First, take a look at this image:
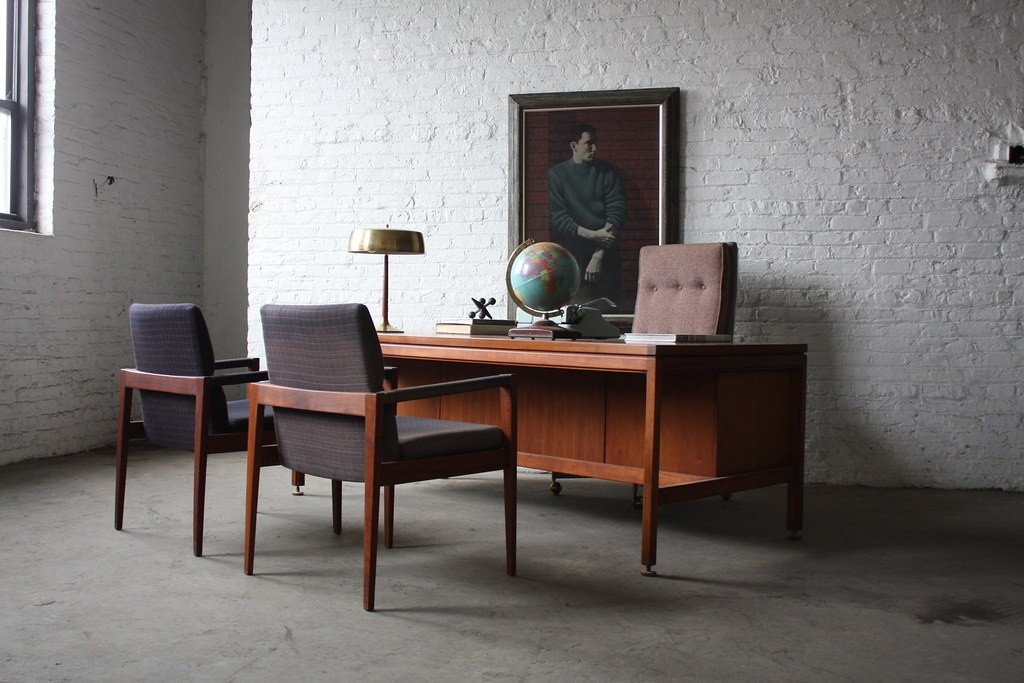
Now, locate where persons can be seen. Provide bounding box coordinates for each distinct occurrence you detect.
[549,124,624,304]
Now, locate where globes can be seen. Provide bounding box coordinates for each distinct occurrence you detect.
[504,236,583,341]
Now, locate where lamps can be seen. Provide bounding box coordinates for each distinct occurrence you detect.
[348,223,425,333]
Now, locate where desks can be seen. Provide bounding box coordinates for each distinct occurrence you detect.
[290,333,808,576]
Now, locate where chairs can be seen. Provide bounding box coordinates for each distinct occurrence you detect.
[244,303,520,611]
[550,242,739,510]
[114,303,342,557]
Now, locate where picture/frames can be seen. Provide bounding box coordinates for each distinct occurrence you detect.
[507,88,681,324]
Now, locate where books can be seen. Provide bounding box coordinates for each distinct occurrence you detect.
[625,333,732,343]
[436,321,519,336]
